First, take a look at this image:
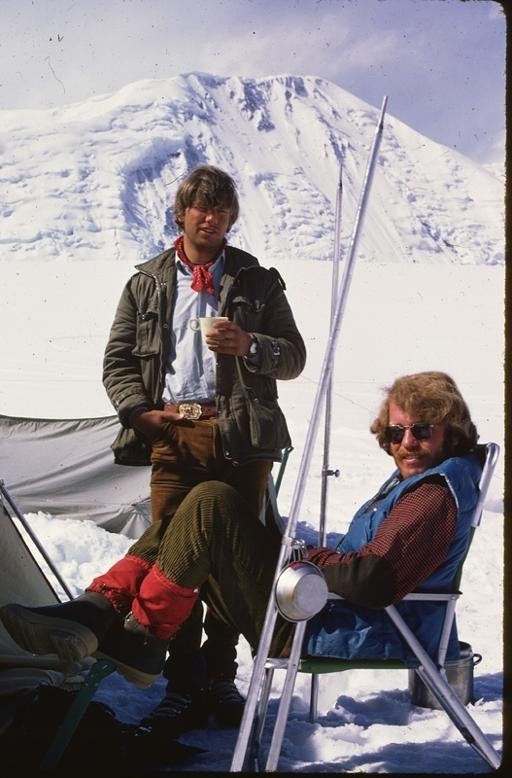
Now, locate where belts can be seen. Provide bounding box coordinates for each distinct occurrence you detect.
[161,402,218,420]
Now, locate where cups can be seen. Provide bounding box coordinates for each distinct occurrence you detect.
[191,316,228,347]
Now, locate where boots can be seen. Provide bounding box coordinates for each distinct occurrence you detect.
[137,660,257,737]
[0,589,171,689]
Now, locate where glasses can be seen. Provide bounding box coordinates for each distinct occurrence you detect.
[385,418,445,443]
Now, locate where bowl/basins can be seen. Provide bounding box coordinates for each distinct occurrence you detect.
[275,560,330,624]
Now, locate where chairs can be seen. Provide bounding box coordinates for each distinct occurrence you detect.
[245,443,504,774]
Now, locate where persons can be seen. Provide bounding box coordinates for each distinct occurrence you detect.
[99,167,311,734]
[1,366,483,689]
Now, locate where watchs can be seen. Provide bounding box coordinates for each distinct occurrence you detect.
[244,333,257,362]
